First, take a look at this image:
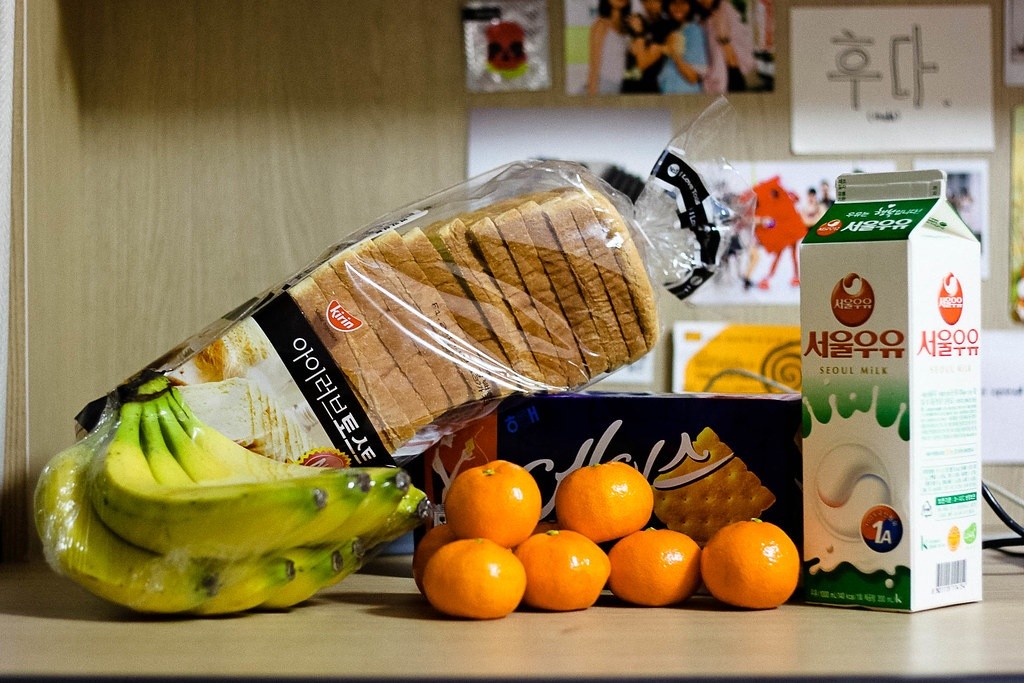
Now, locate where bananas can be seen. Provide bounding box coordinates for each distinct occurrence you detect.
[31,370,433,617]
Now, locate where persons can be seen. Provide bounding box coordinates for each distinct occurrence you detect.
[801,180,834,228]
[589,0,762,95]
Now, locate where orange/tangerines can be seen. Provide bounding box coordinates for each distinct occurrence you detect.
[412,460,800,620]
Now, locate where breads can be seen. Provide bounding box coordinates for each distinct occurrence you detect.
[72,187,662,467]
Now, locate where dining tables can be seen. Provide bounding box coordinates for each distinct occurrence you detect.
[2,463,1022,681]
